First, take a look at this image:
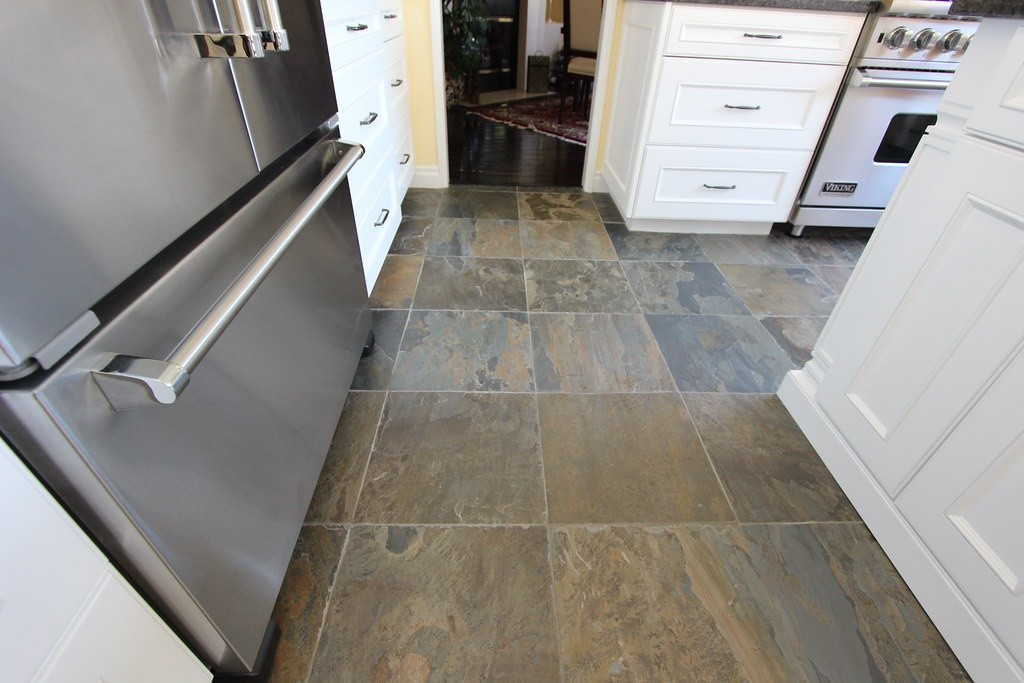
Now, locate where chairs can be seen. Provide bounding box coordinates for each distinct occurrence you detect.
[558,0,604,125]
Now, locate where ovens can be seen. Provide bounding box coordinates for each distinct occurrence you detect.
[799,68,956,212]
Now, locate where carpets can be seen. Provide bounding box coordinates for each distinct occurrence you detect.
[467,94,588,148]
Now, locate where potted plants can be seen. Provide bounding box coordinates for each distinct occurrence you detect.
[441,0,488,112]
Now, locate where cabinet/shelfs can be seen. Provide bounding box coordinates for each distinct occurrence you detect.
[0,436,215,683]
[605,0,869,235]
[321,0,414,297]
[774,17,1024,683]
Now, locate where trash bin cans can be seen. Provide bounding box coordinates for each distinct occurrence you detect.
[528,55,550,92]
